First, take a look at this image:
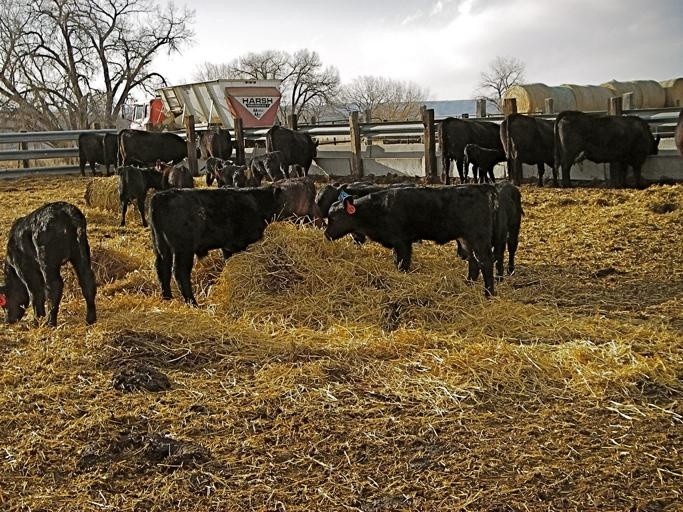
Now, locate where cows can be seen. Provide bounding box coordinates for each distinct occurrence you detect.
[316,178,522,298]
[77,126,325,228]
[437,116,505,185]
[675,109,683,157]
[147,188,312,309]
[463,143,509,185]
[499,112,562,188]
[552,110,661,187]
[1,202,97,326]
